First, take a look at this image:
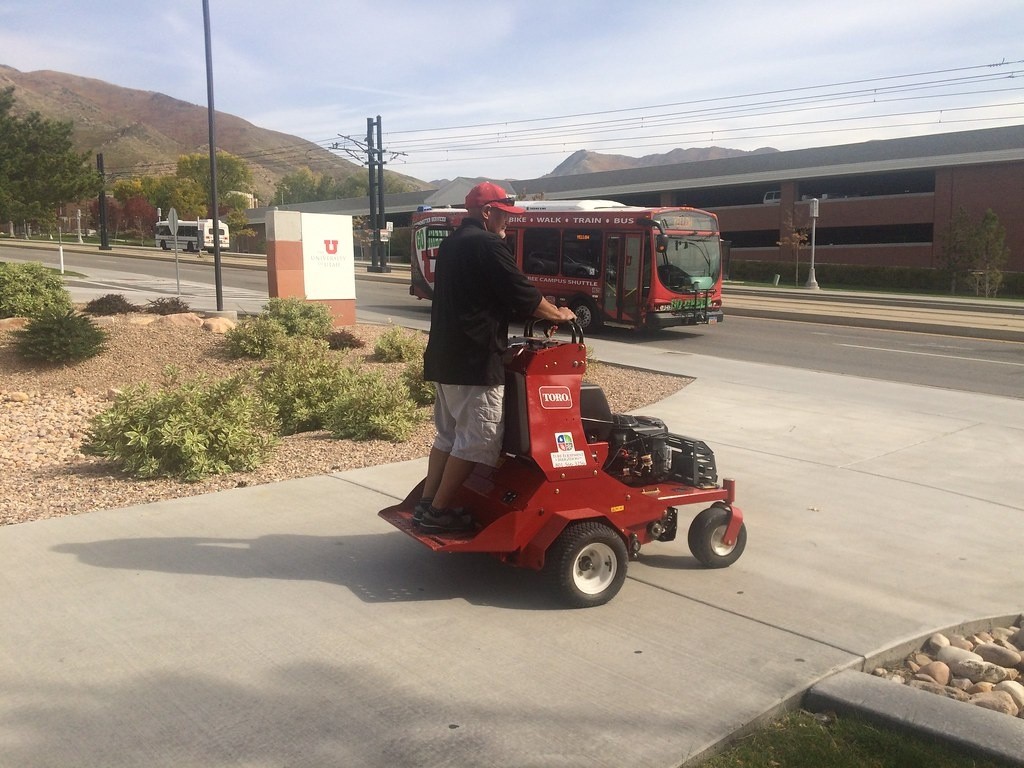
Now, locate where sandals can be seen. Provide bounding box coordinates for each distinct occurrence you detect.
[411,499,432,525]
[418,507,476,532]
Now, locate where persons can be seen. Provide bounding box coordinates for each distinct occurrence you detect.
[413,181,576,533]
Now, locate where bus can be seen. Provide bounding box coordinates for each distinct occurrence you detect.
[155,218,231,251]
[408,198,732,335]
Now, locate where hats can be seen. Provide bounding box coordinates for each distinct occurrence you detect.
[465,181,526,214]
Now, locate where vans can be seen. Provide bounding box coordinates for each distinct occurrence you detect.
[764,190,780,205]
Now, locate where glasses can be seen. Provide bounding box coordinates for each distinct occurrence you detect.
[482,196,516,209]
